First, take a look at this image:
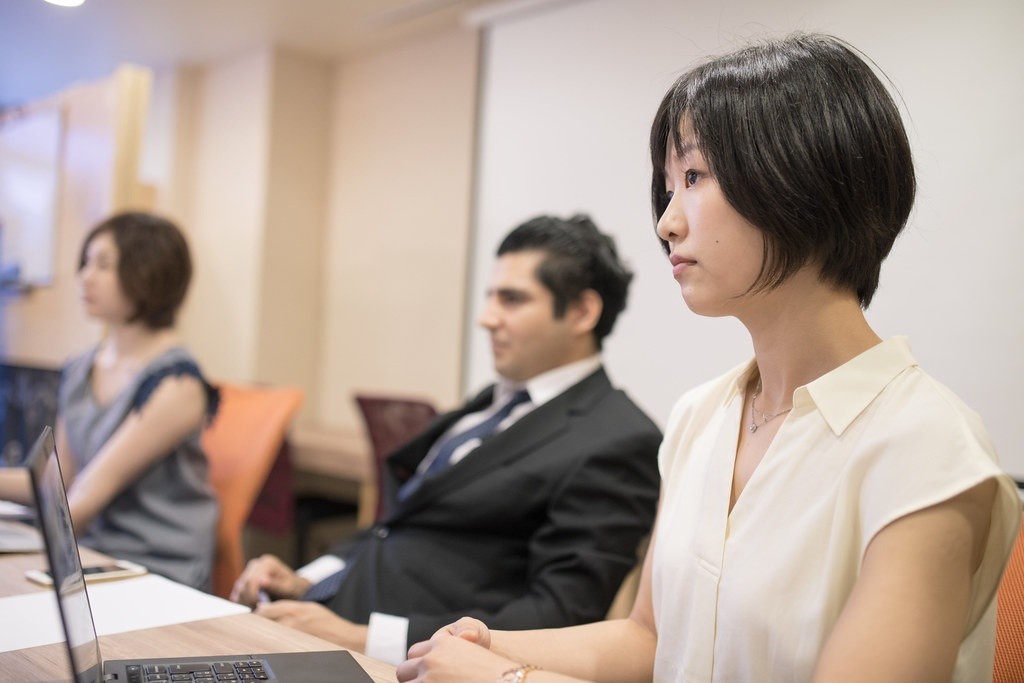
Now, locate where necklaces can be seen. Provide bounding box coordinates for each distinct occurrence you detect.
[750,374,792,434]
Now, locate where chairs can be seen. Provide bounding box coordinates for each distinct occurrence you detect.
[288,369,380,532]
[193,377,303,601]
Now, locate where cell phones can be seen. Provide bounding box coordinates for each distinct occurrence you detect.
[26,560,148,585]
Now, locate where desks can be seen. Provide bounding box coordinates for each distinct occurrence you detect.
[0,523,406,683]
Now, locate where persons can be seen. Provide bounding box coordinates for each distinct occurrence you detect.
[232,35,1019,683]
[0,213,223,588]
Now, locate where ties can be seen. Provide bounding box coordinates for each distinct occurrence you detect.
[301,390,530,601]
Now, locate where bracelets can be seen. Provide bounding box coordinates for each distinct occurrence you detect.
[503,664,543,683]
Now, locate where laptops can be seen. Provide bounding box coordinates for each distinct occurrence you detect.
[26,424,374,683]
